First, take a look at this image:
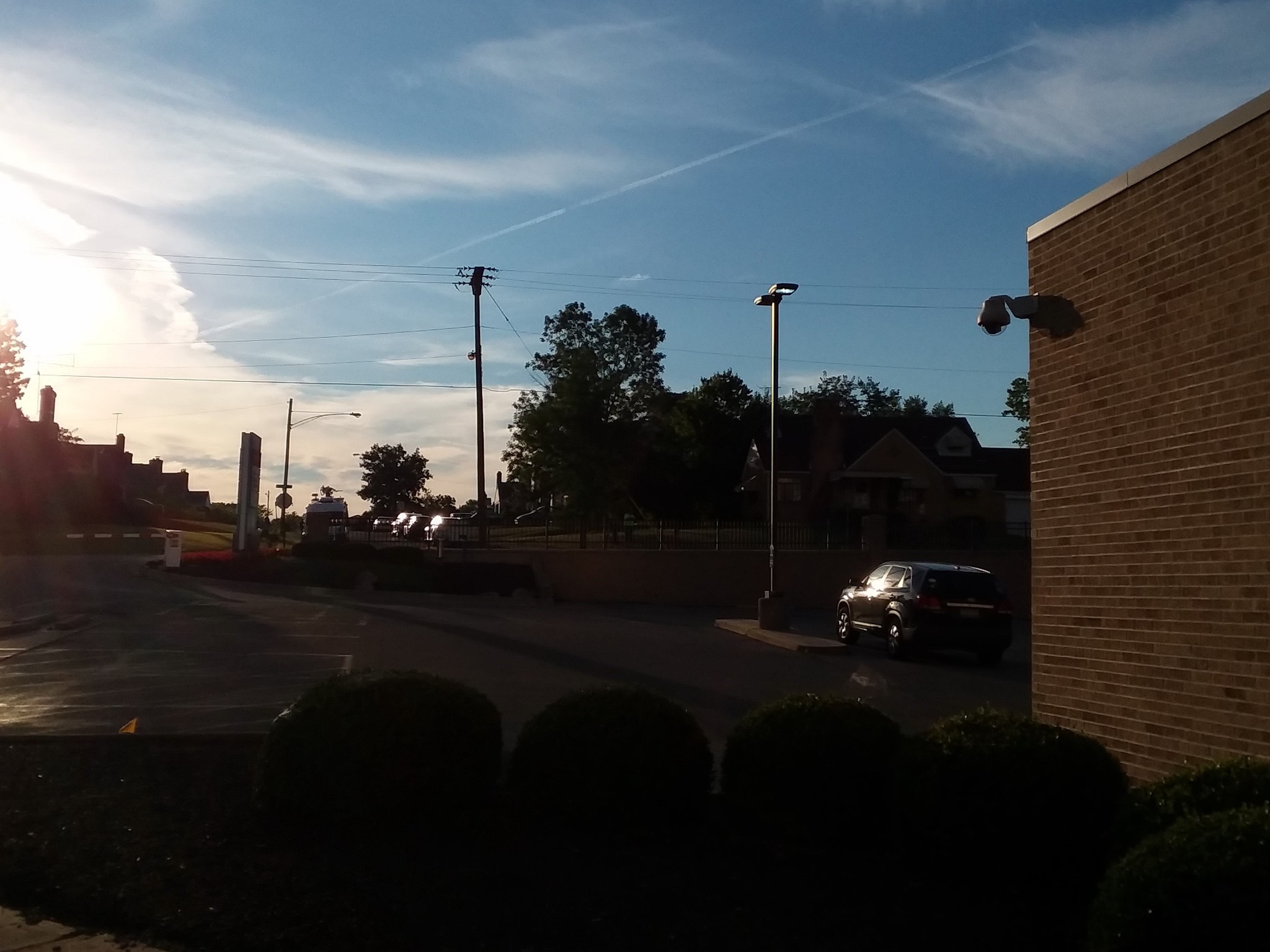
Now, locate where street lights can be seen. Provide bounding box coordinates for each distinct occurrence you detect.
[265,490,269,517]
[753,282,801,631]
[280,398,363,547]
[467,351,489,546]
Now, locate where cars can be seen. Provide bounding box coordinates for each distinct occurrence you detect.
[126,497,165,525]
[513,505,568,526]
[373,512,505,546]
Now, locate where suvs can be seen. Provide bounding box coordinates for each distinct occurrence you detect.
[836,559,1014,667]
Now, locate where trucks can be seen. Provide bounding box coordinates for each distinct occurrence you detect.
[303,498,350,545]
